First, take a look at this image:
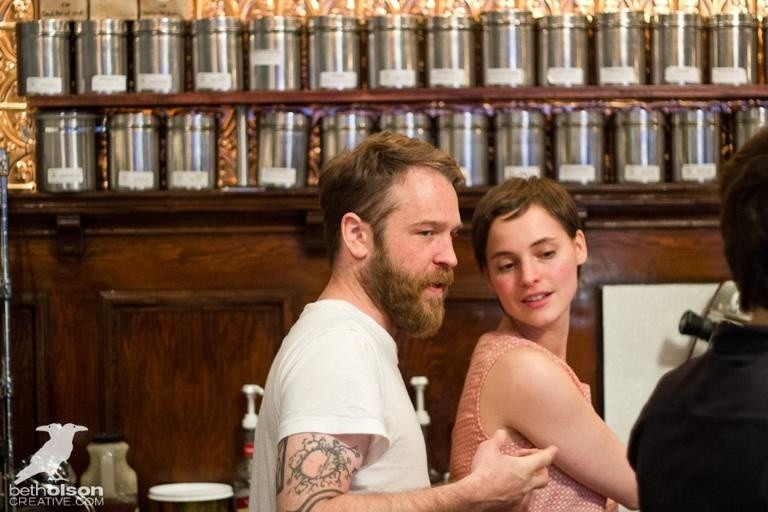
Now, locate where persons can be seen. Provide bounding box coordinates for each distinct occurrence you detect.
[625,113,768,512]
[448,175,638,512]
[249,130,558,512]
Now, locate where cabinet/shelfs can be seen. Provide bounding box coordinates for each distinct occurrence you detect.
[0,201,334,511]
[334,197,733,512]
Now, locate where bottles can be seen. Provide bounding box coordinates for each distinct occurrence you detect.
[232,444,254,512]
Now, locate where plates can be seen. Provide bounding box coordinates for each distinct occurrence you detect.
[148,482,234,497]
[147,493,234,503]
